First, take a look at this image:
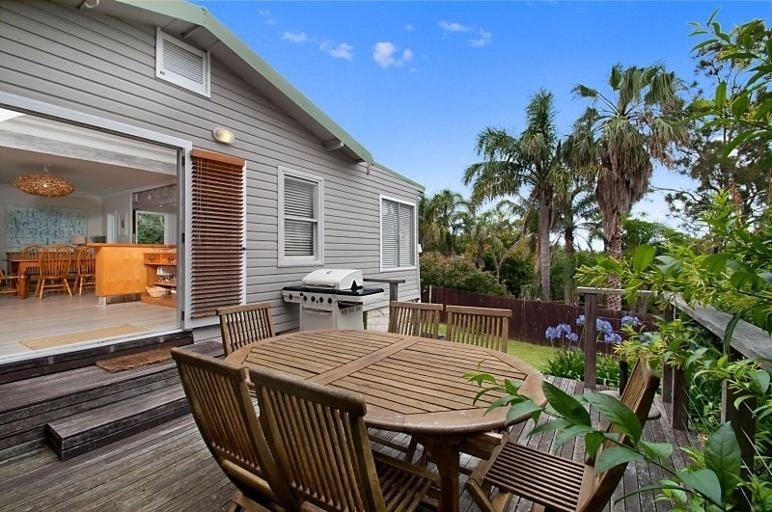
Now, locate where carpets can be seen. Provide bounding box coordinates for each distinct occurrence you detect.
[95,345,180,373]
[21,321,146,351]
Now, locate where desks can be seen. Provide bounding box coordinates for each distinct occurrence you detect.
[220,327,551,511]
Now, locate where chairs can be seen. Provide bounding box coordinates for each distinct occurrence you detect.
[216,301,275,356]
[461,354,661,510]
[0,240,103,303]
[249,365,441,511]
[445,306,512,354]
[170,345,324,512]
[388,300,444,339]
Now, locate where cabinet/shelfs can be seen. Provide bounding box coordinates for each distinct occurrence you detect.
[89,239,176,311]
[142,253,176,307]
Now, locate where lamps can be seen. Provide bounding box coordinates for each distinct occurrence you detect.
[11,163,75,200]
[213,125,237,145]
